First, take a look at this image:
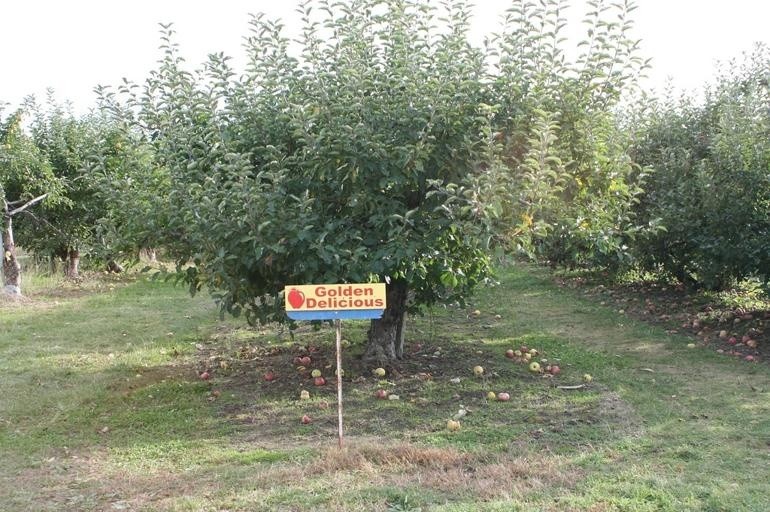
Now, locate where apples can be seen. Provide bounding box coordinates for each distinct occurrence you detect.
[586,284,769,362]
[200,309,592,430]
[289,289,305,309]
